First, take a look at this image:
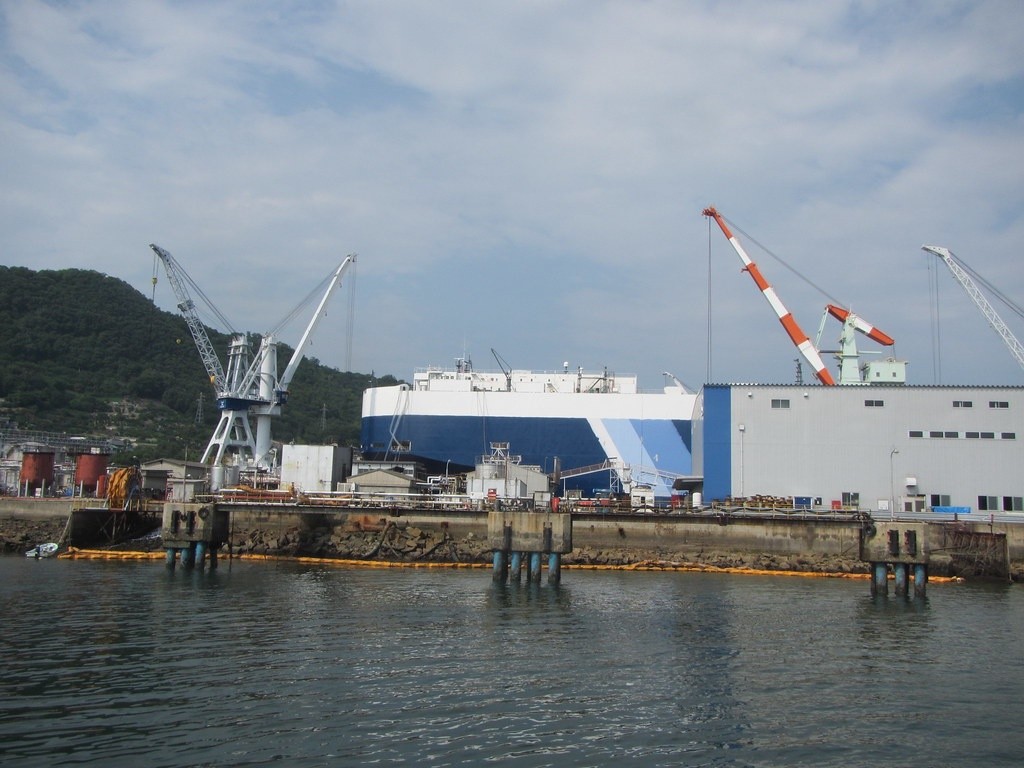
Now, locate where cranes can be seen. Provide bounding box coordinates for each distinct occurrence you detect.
[923,243,1024,385]
[703,206,895,384]
[150,243,358,467]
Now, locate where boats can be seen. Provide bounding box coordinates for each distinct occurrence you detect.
[26,542,58,558]
